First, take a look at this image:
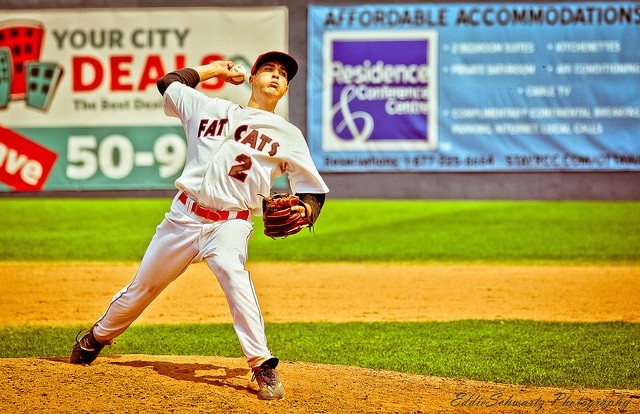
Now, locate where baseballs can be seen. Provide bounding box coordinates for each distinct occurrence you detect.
[230,65,246,83]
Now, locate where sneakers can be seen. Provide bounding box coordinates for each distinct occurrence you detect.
[251,357,285,399]
[70,328,104,364]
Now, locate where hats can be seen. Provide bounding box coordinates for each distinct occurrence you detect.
[251,50,299,81]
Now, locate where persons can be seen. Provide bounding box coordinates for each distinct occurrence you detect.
[70,50,329,400]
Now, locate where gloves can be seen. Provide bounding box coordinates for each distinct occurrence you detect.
[263,198,310,236]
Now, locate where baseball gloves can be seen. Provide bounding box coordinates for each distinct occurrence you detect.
[262,193,309,239]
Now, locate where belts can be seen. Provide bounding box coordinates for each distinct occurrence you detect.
[178,191,249,221]
